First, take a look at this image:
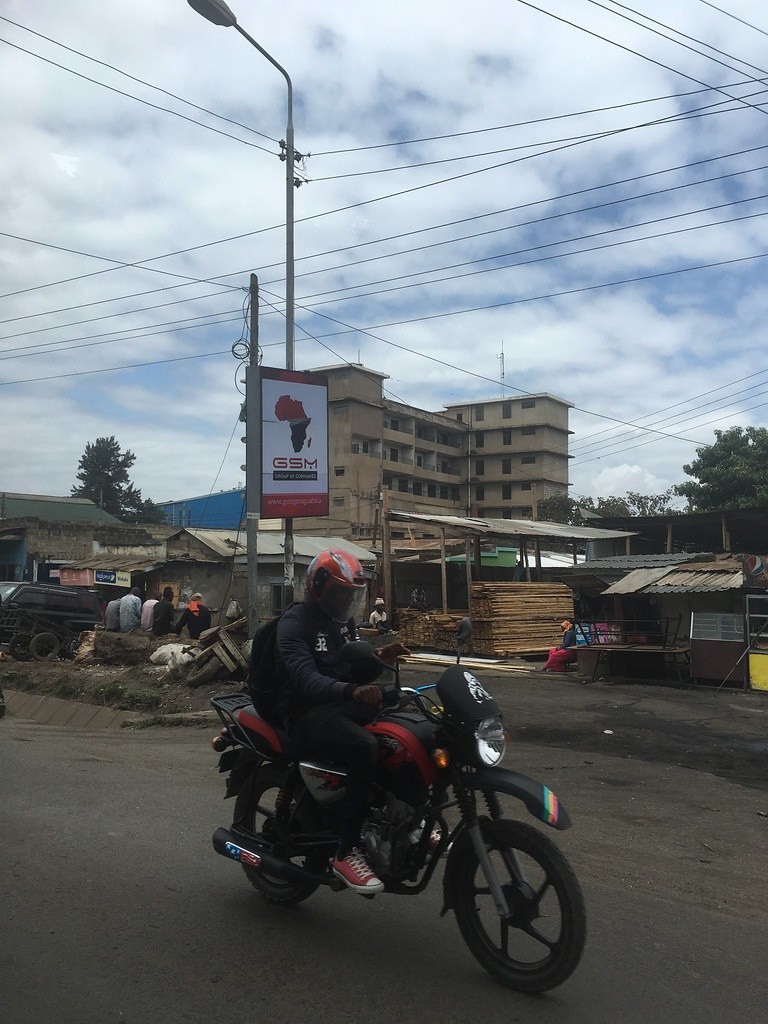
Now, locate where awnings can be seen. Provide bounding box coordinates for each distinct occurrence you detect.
[600,567,744,594]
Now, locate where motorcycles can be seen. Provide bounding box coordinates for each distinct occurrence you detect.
[211,617,587,995]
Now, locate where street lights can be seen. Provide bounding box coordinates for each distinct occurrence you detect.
[187,0,297,613]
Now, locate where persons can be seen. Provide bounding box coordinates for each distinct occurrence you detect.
[120,587,141,630]
[153,587,176,636]
[370,598,386,628]
[141,590,162,630]
[175,593,211,639]
[277,550,455,894]
[106,589,129,631]
[541,621,575,672]
[410,584,432,612]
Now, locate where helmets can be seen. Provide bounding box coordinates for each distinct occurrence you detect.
[304,549,365,603]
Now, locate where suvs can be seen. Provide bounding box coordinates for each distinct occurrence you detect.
[0,580,102,649]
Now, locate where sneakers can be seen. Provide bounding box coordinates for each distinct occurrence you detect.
[332,847,384,894]
[410,827,457,859]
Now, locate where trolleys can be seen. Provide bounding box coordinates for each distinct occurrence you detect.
[0,599,82,663]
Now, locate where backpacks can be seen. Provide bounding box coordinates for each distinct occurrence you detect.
[248,600,305,722]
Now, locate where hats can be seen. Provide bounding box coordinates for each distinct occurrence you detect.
[190,593,203,601]
[374,597,385,606]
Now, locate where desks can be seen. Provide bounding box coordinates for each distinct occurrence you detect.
[564,613,690,683]
